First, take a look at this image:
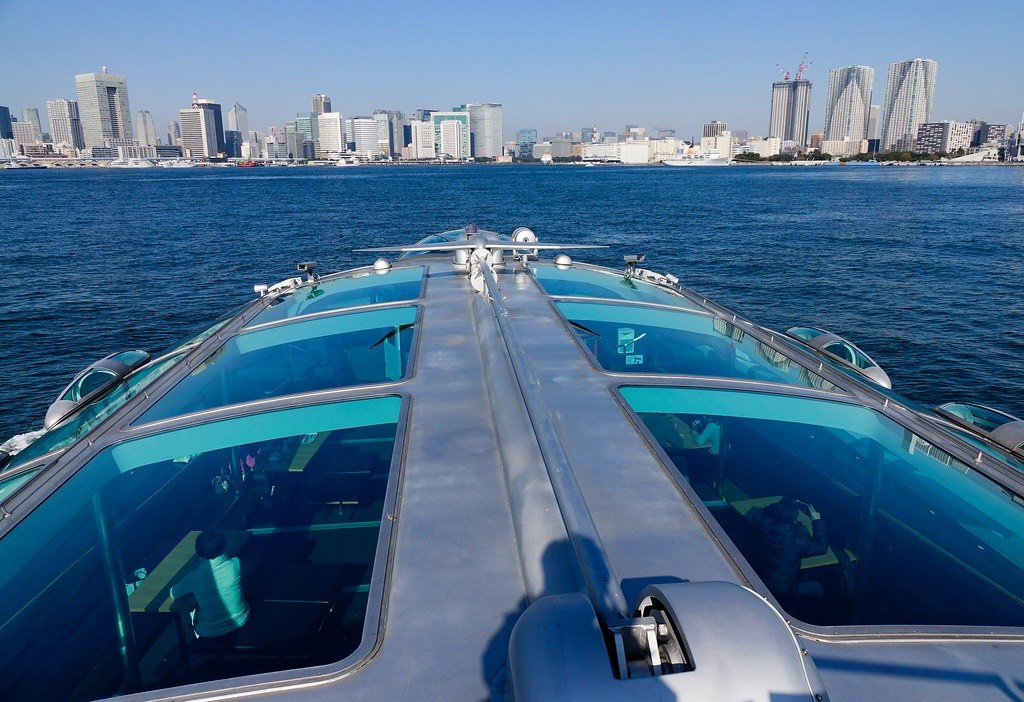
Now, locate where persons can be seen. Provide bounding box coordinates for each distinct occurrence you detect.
[642,413,690,482]
[746,495,830,608]
[692,414,736,481]
[228,443,273,500]
[168,528,253,642]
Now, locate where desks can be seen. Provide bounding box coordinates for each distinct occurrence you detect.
[127,530,201,652]
[728,495,838,570]
[637,412,713,449]
[253,430,333,521]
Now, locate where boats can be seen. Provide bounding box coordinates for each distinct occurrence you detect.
[0,213,1022,702]
[237,161,263,168]
[659,156,733,167]
[104,157,157,168]
[158,160,198,168]
